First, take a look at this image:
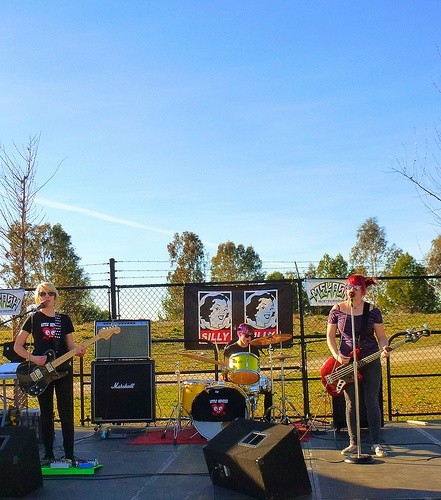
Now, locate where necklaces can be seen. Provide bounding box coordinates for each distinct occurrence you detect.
[354,301,362,309]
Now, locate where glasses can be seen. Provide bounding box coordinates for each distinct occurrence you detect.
[39,291,56,297]
[345,283,363,290]
[240,332,255,339]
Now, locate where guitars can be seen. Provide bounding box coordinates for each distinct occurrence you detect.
[321,324,432,398]
[16,325,121,396]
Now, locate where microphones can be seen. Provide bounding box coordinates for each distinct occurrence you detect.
[348,290,355,298]
[34,301,47,311]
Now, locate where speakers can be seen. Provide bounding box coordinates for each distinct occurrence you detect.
[332,377,384,431]
[0,427,44,498]
[202,417,313,500]
[90,359,155,423]
[93,320,151,360]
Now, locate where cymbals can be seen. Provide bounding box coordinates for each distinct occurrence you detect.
[267,355,299,358]
[179,354,229,367]
[250,334,293,345]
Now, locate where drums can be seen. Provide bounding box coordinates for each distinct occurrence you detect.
[181,379,217,420]
[227,352,260,385]
[191,381,253,441]
[245,376,272,394]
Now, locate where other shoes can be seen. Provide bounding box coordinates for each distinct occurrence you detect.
[41,454,55,460]
[65,456,75,462]
[341,445,356,456]
[372,443,387,457]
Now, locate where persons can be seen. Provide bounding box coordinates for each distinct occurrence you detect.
[327,274,390,458]
[13,282,86,465]
[223,323,273,422]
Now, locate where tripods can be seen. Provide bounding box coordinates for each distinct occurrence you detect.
[259,343,301,434]
[271,343,311,430]
[160,364,194,445]
